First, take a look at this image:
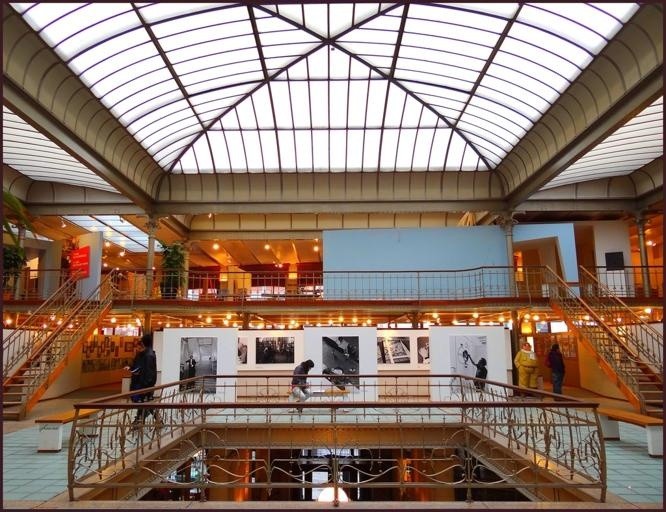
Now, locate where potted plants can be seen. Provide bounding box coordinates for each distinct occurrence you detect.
[148,236,192,299]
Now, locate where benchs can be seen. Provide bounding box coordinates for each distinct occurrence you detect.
[591,407,666,458]
[35,408,104,453]
[539,281,594,298]
[287,388,350,413]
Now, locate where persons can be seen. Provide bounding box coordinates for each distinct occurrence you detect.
[123,340,152,421]
[131,334,167,430]
[289,358,315,414]
[466,353,489,402]
[185,354,196,386]
[548,343,567,402]
[514,341,542,394]
